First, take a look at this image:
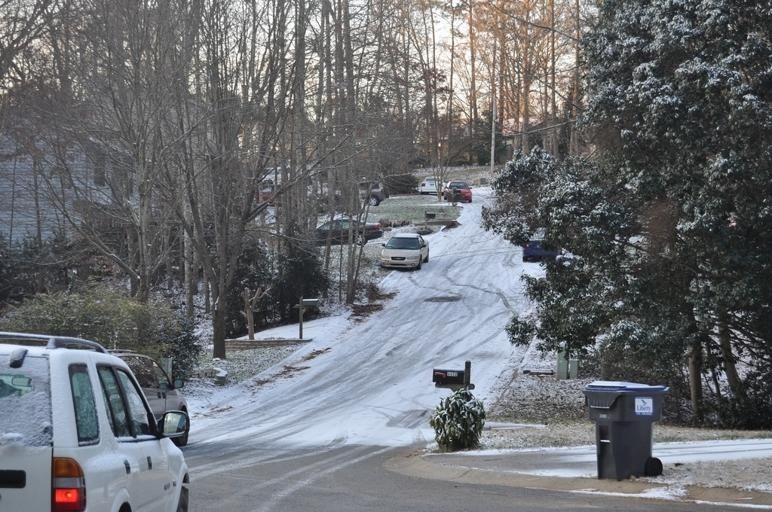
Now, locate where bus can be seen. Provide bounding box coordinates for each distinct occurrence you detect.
[251,166,330,206]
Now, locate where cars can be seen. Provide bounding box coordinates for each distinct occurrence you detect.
[444,180,474,203]
[317,218,384,245]
[381,232,430,270]
[523,226,561,262]
[105,349,190,447]
[420,176,446,196]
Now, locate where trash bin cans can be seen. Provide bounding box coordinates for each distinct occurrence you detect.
[582,380,671,481]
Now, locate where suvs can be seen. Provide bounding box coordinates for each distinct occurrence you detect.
[336,180,387,207]
[0,331,191,512]
